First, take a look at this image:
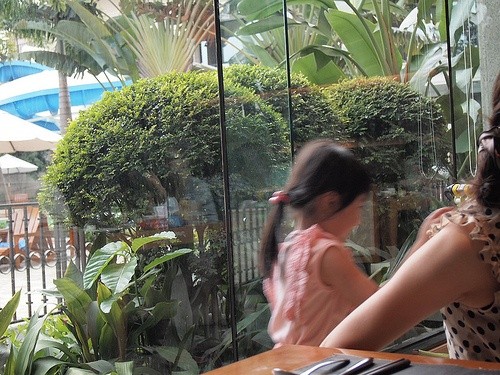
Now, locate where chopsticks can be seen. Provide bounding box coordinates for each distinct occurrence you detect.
[362,357,410,375]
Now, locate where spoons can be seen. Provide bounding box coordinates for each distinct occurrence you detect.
[271,358,350,375]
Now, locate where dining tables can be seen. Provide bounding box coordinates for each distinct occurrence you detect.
[200,344,500,375]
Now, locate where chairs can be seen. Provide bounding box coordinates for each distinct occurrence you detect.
[0,206,55,262]
[12,194,28,212]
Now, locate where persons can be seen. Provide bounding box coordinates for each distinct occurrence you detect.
[258,139,452,348]
[317,72,499,363]
